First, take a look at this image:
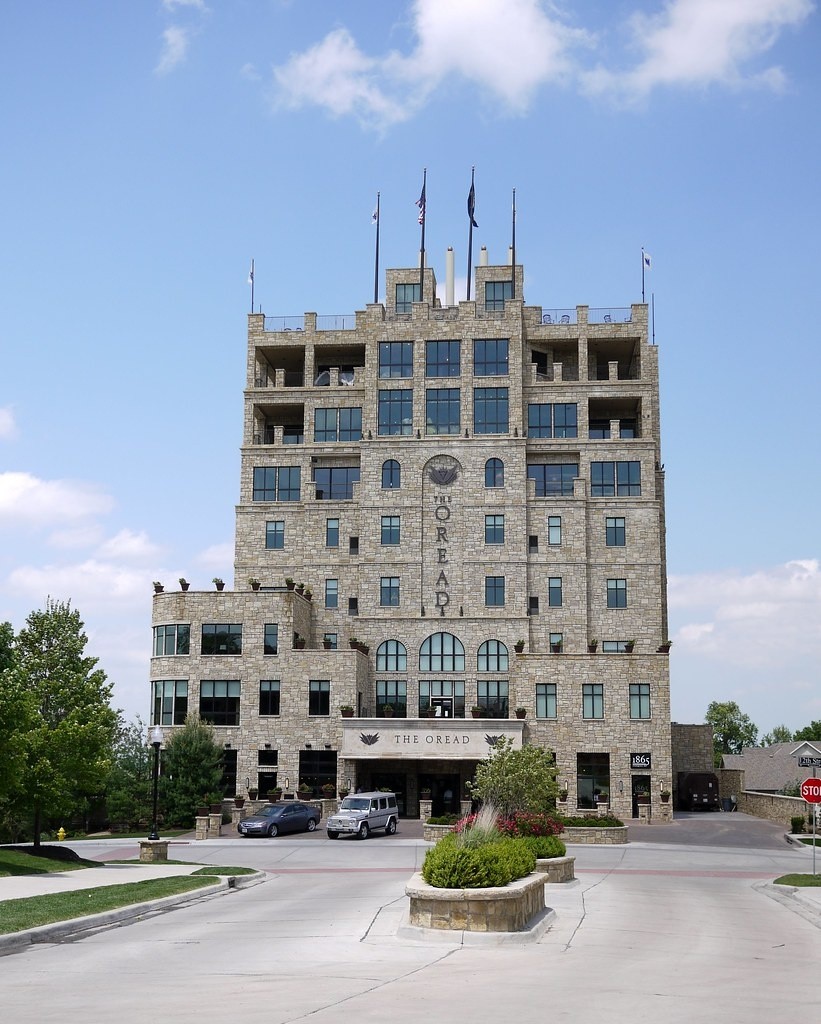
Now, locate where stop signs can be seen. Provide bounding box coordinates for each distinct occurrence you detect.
[801,778,821,804]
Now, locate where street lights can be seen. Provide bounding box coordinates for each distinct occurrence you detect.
[148,724,164,840]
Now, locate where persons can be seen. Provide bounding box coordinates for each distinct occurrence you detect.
[443,785,452,814]
[356,782,380,794]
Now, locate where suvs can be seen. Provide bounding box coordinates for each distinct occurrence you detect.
[688,782,720,812]
[326,792,399,839]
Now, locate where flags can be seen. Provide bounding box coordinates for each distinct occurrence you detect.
[248,262,255,283]
[371,197,378,225]
[415,183,427,224]
[644,252,651,271]
[467,175,479,227]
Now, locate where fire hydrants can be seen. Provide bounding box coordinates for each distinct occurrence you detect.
[56,827,66,841]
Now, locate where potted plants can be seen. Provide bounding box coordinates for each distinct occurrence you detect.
[383,704,394,718]
[463,790,470,801]
[427,706,437,718]
[273,787,283,800]
[339,789,349,800]
[295,583,305,595]
[302,786,313,801]
[285,577,296,590]
[197,802,209,816]
[658,639,673,653]
[660,790,671,802]
[208,791,224,814]
[322,784,336,799]
[267,790,279,803]
[233,794,245,808]
[558,790,569,802]
[248,577,261,591]
[349,637,370,655]
[421,788,431,799]
[321,637,332,649]
[515,707,527,719]
[248,788,259,800]
[552,640,563,653]
[514,640,525,652]
[212,578,226,591]
[178,578,191,591]
[587,639,598,652]
[638,792,650,804]
[296,783,307,799]
[295,637,306,649]
[153,582,164,593]
[623,639,637,653]
[471,706,482,718]
[340,705,354,717]
[598,790,609,803]
[303,589,312,601]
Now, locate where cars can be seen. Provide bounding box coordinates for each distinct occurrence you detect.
[237,802,322,838]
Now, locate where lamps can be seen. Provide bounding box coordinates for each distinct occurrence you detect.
[246,776,249,789]
[660,781,663,792]
[620,781,623,792]
[564,780,568,791]
[286,778,289,789]
[347,779,351,790]
